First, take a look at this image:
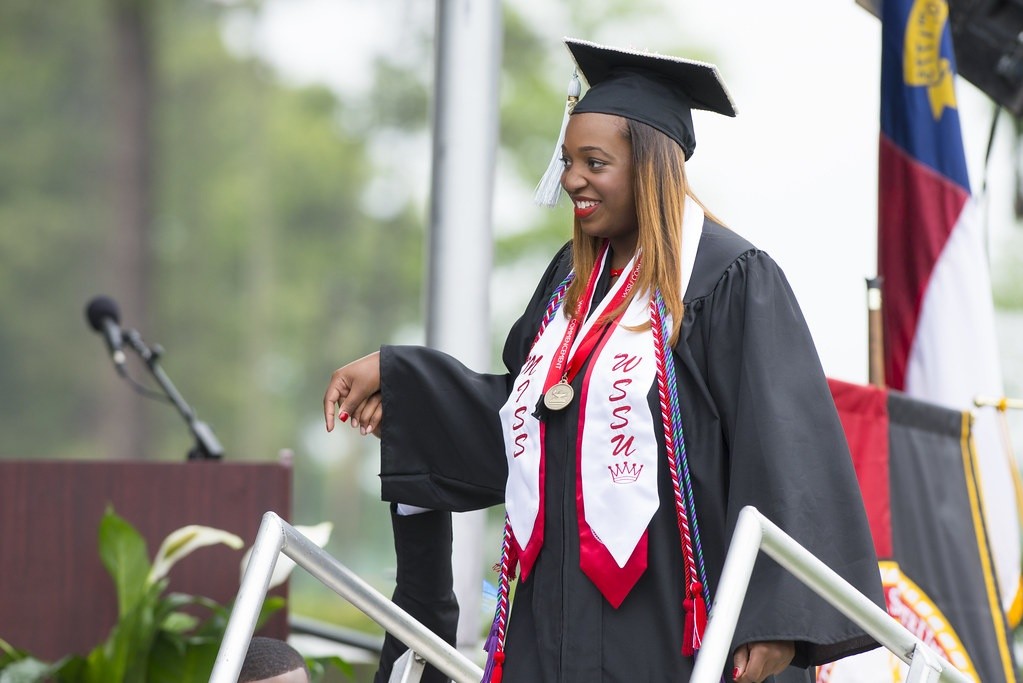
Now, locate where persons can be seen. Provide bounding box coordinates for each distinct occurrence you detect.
[237,391,459,683]
[322,36,888,683]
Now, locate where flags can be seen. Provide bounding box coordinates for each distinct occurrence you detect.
[877,0,1023,629]
[815,377,1023,683]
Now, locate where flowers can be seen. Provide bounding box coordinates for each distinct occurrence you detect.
[0,500,334,683]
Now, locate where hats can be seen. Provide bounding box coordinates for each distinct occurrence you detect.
[533,36,738,208]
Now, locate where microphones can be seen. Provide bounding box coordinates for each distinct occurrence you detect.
[85,294,124,355]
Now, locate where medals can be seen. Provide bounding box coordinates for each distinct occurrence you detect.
[544,383,574,411]
